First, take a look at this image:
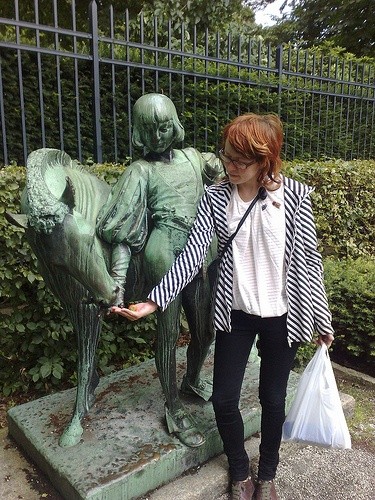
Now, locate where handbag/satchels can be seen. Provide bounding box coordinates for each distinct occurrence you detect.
[206,258,218,295]
[281,342,352,450]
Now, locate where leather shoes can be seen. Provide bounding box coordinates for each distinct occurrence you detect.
[230,478,255,500]
[256,477,278,500]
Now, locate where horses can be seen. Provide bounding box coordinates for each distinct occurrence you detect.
[3,148,219,447]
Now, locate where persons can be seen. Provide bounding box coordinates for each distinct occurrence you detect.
[106,112,335,500]
[95,92,228,447]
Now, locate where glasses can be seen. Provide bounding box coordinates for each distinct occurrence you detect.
[218,147,257,170]
